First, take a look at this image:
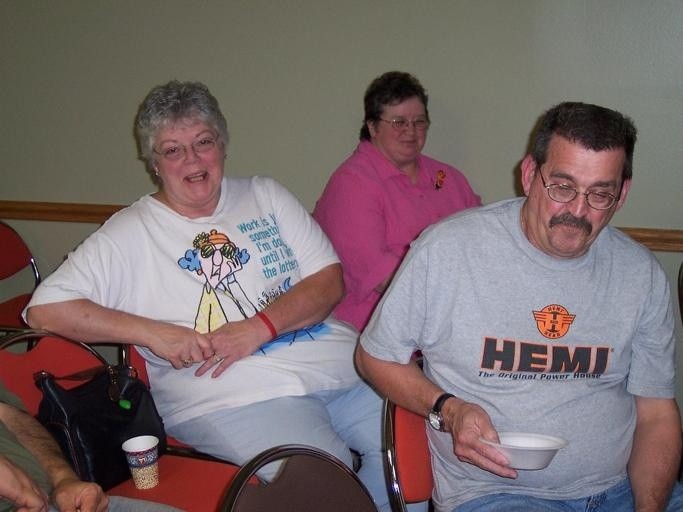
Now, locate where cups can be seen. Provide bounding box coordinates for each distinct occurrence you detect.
[121,434,160,491]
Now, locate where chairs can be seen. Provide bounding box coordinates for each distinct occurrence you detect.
[119,345,211,460]
[0,333,258,512]
[381,358,437,512]
[221,445,379,512]
[0,222,43,352]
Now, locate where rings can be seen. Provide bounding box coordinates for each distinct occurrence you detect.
[182,358,192,364]
[212,353,221,361]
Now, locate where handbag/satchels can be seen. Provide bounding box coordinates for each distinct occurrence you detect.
[32,364,167,493]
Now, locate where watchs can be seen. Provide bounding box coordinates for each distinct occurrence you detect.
[429,392,456,433]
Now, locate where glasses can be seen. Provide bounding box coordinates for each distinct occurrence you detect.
[152,134,219,161]
[537,167,621,211]
[378,117,430,132]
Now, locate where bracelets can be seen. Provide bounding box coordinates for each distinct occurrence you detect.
[254,311,276,343]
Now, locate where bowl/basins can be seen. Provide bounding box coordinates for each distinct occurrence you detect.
[478,431,567,472]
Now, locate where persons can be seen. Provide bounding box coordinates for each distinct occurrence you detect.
[0,385,187,512]
[21,76,429,512]
[300,69,478,330]
[351,99,683,512]
